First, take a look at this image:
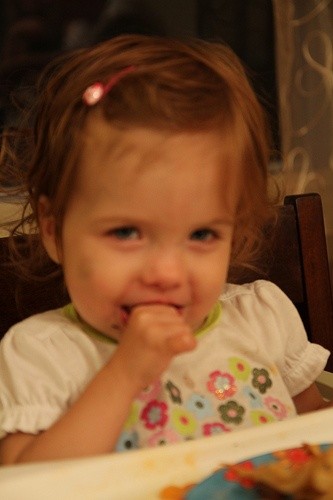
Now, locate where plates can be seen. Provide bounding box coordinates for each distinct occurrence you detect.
[182,443,333,500]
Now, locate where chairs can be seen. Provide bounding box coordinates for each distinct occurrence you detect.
[0,190,333,413]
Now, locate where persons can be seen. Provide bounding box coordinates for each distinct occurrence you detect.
[1,33,333,500]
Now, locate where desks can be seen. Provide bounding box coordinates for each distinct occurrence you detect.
[0,389,332,500]
